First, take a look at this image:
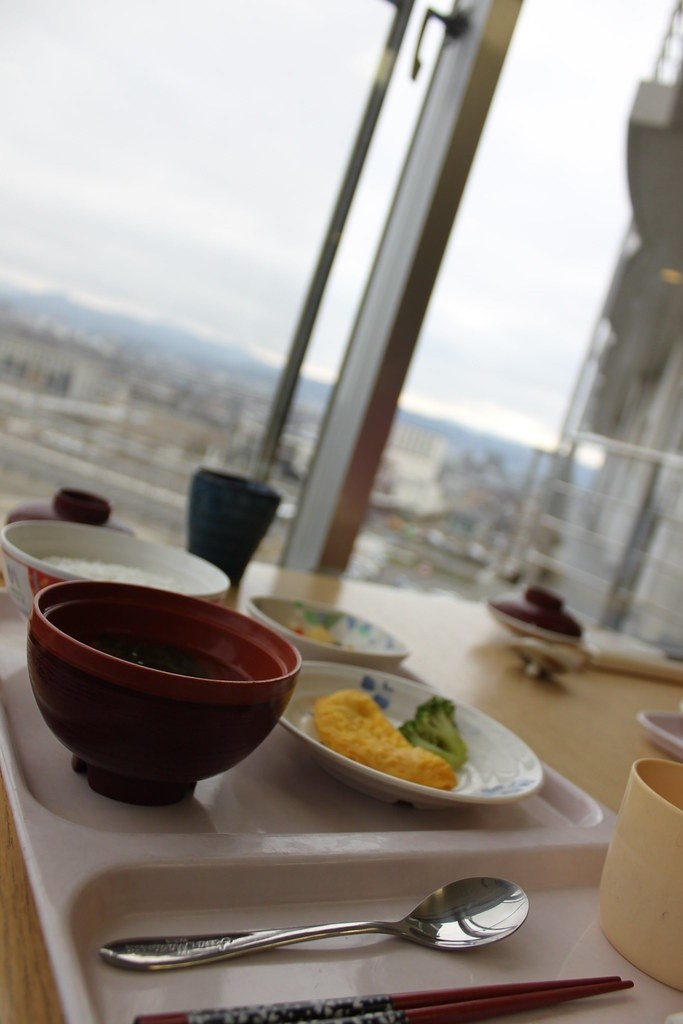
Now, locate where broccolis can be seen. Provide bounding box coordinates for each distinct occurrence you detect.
[400,697,468,768]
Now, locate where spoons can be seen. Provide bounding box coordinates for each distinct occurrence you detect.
[98,875,531,972]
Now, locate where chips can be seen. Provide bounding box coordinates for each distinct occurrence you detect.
[315,690,457,788]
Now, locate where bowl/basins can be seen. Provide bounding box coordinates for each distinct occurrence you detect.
[0,520,233,633]
[246,592,409,674]
[26,579,304,807]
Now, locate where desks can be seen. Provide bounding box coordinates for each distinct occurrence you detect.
[0,551,683,1024]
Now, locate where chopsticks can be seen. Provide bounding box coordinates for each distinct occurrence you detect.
[133,975,636,1024]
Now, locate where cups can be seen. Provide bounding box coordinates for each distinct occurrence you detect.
[597,756,683,993]
[188,464,282,588]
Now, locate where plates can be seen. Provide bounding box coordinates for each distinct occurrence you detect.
[636,708,683,760]
[277,659,546,812]
